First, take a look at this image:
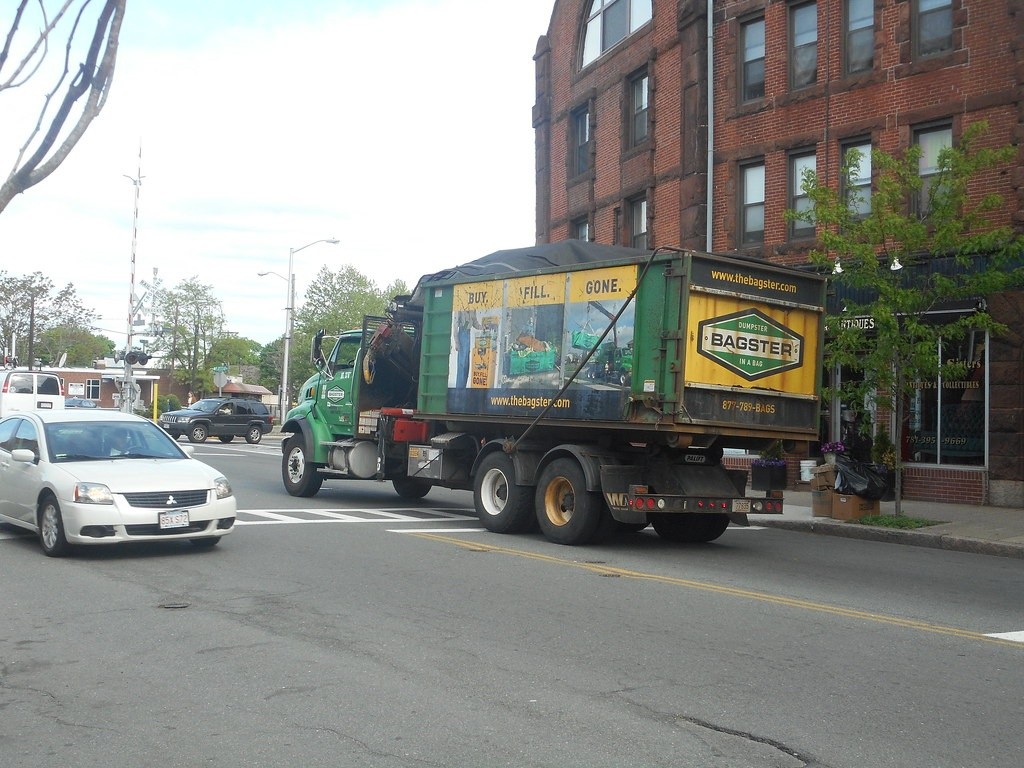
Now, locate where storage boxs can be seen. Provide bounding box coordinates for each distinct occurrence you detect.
[809,466,880,521]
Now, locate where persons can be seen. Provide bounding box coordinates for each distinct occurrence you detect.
[109,428,127,456]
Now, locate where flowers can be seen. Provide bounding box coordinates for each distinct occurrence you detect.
[751,459,787,468]
[821,441,846,453]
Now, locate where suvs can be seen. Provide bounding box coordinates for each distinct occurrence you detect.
[157,398,273,445]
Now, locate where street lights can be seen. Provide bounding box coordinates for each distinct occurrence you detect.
[258,239,339,424]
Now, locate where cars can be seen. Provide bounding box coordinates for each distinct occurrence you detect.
[1,367,65,420]
[0,412,238,557]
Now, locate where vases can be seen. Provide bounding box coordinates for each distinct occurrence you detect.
[825,453,835,464]
[752,466,788,491]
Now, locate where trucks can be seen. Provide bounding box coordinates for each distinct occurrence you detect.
[281,235,821,544]
